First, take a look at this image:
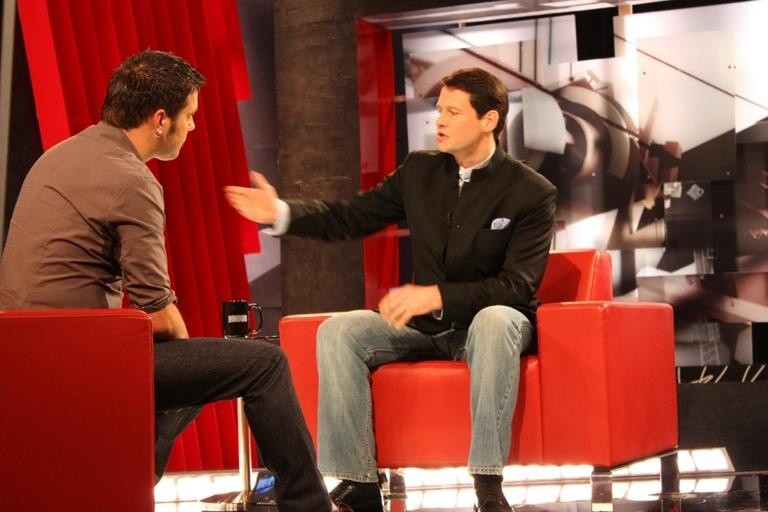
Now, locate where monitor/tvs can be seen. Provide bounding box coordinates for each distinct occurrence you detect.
[390,1,768,388]
[249,469,278,506]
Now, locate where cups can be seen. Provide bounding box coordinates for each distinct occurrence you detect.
[222,299,264,340]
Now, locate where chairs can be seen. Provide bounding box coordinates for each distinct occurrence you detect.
[275,250,685,510]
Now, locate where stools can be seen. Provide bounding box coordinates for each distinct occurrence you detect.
[0,300,158,512]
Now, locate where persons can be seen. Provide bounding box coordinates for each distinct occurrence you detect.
[0,49,334,511]
[223,65,558,512]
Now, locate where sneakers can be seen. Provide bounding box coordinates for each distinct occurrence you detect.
[329,477,383,512]
[471,483,514,512]
